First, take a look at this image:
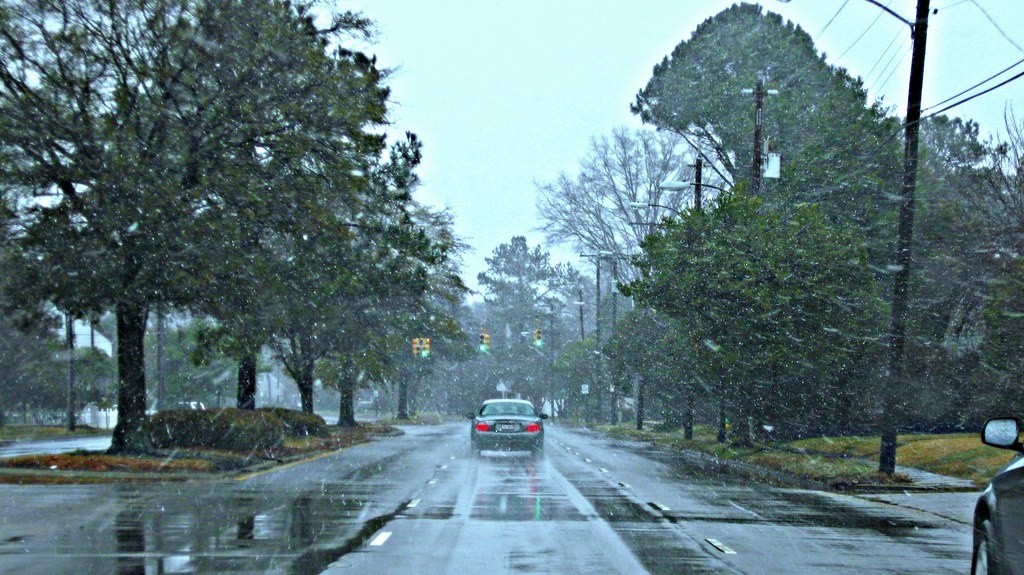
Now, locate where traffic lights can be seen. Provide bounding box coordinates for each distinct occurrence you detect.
[412,339,419,356]
[532,329,542,348]
[483,334,491,350]
[479,335,485,352]
[416,339,427,358]
[424,339,430,356]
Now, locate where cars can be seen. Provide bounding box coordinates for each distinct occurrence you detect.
[970,415,1024,575]
[465,398,548,460]
